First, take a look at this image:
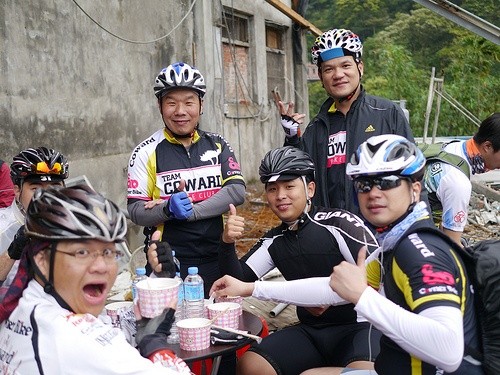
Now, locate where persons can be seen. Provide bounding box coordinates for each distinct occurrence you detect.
[208,134,481,375]
[213,146,380,375]
[0,160,15,208]
[0,147,70,288]
[0,184,195,375]
[277,29,416,232]
[414,110,500,250]
[127,62,246,299]
[460,240,500,375]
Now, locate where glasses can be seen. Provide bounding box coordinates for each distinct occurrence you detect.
[352,174,409,193]
[45,246,124,262]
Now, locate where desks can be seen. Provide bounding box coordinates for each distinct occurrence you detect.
[105,299,263,375]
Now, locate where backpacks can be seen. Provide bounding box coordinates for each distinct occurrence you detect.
[384,222,500,375]
[416,140,470,179]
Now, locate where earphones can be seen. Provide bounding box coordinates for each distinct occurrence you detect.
[412,191,417,202]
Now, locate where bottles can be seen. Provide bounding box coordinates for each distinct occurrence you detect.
[133,268,148,302]
[172,250,180,277]
[184,267,204,319]
[166,272,184,344]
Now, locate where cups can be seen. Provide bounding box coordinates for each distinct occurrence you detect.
[203,298,213,318]
[176,319,211,350]
[208,303,241,331]
[215,295,242,315]
[105,302,132,321]
[136,278,179,318]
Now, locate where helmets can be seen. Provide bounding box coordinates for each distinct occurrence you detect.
[259,146,316,176]
[25,185,128,242]
[346,134,427,175]
[10,147,69,178]
[153,63,206,98]
[312,28,363,63]
